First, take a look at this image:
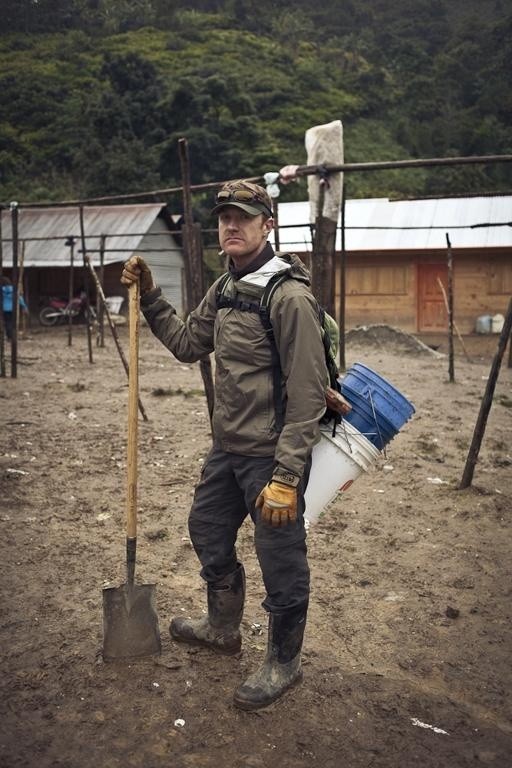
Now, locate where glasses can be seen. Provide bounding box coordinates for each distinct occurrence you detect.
[216,190,254,203]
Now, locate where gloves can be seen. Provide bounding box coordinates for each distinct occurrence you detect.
[254,481,297,528]
[119,255,153,297]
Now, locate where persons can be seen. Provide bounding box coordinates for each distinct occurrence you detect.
[2,275,30,343]
[119,180,331,710]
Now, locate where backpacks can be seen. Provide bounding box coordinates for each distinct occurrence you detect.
[214,252,342,425]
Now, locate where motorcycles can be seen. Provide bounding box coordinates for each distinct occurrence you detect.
[38,292,96,326]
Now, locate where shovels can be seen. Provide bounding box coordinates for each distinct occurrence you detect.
[102,267,161,659]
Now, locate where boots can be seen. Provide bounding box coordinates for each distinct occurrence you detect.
[232,602,308,711]
[168,562,244,655]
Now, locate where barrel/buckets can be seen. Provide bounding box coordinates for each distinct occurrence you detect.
[107,296,125,314]
[340,362,418,454]
[302,414,380,531]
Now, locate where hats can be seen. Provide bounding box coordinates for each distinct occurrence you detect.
[209,180,272,218]
